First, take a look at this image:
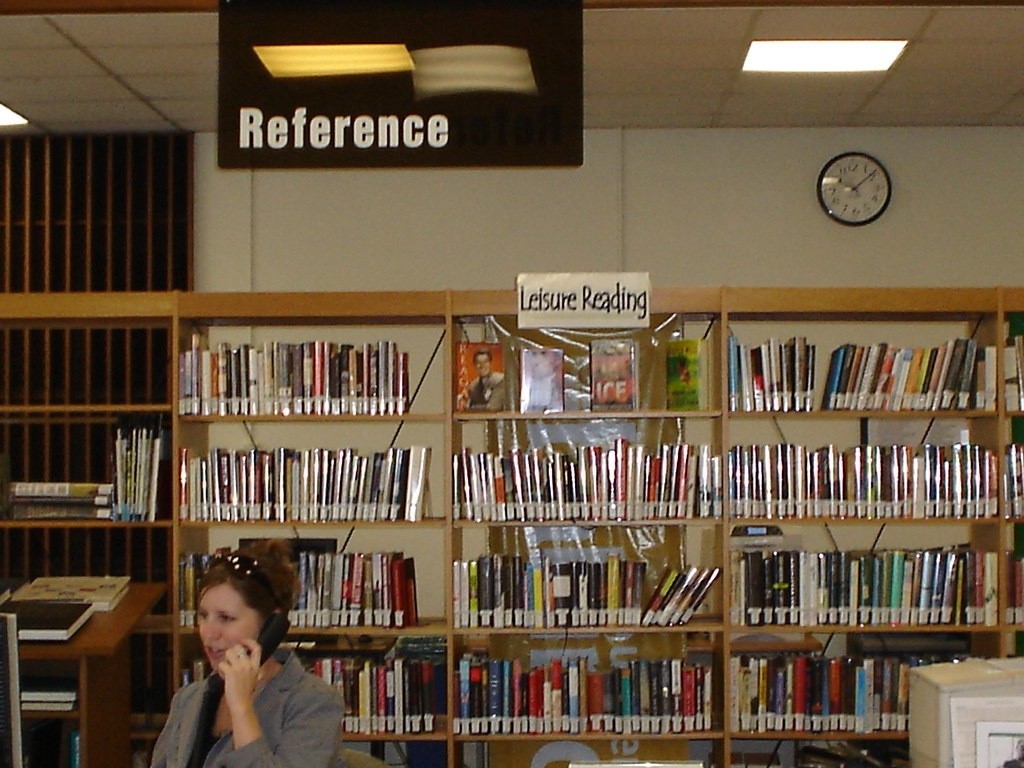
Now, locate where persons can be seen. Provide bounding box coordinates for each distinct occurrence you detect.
[458,347,505,410]
[146,534,345,768]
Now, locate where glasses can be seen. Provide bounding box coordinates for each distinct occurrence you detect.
[206,553,275,599]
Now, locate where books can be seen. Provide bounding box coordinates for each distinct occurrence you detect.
[0,572,133,641]
[9,417,165,522]
[451,434,721,521]
[177,331,413,417]
[457,633,718,735]
[729,522,1024,626]
[347,741,492,768]
[178,442,431,521]
[725,315,1023,414]
[181,646,448,734]
[730,435,1023,518]
[730,629,981,768]
[455,333,712,412]
[16,674,80,767]
[177,535,420,628]
[454,549,724,626]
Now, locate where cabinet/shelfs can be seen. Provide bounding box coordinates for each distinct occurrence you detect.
[0,580,168,768]
[2,287,1024,768]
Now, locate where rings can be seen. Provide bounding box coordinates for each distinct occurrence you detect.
[238,648,247,659]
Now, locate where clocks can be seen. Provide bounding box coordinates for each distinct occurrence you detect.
[817,152,892,225]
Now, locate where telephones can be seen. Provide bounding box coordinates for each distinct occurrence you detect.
[209,613,291,694]
